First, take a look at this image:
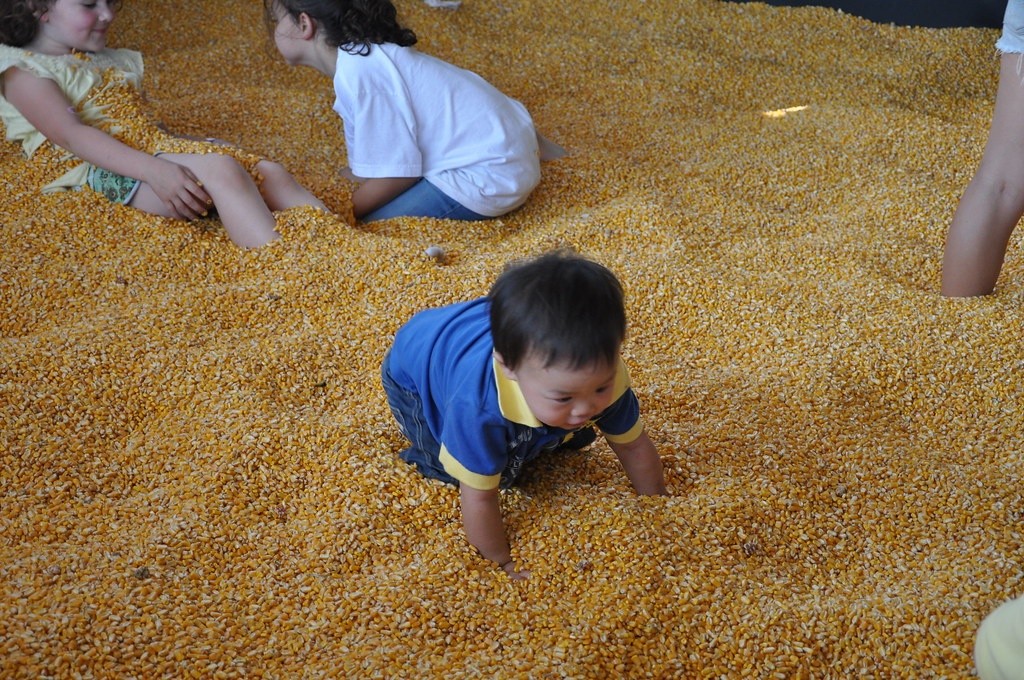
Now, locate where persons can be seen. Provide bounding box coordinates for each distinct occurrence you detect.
[0,0,333,250]
[381,253,670,578]
[262,0,542,226]
[938,0,1024,298]
